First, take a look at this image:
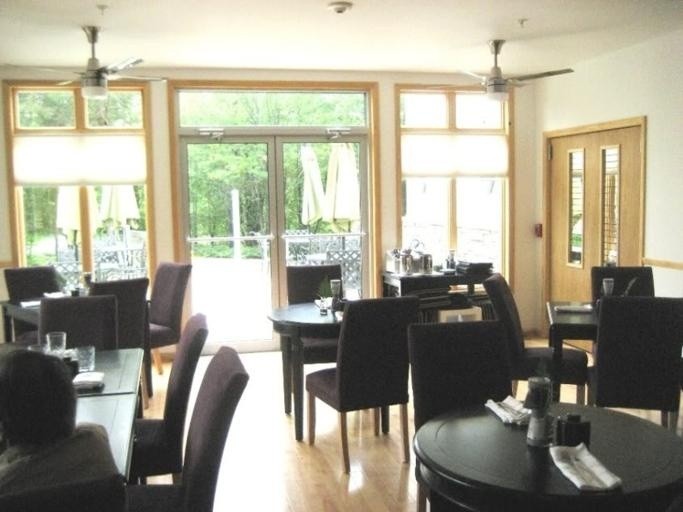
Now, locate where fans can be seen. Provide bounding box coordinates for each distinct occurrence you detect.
[426,38,574,91]
[34,25,166,87]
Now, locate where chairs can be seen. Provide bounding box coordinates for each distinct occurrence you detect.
[42,294,118,350]
[131,317,207,485]
[84,275,149,382]
[149,264,190,396]
[5,267,59,342]
[125,346,250,512]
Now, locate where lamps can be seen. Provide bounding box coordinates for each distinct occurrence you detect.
[81,76,109,100]
[486,84,509,99]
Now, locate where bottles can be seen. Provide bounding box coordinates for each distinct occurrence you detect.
[320,295,328,317]
[596,278,615,319]
[444,249,456,274]
[556,411,592,451]
[330,280,343,313]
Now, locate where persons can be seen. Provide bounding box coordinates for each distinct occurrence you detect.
[0,349,118,498]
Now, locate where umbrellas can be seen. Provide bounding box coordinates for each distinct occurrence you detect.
[55,185,101,264]
[101,183,139,242]
[324,144,360,232]
[300,141,324,224]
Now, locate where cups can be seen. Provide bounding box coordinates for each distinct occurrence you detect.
[28,331,96,372]
